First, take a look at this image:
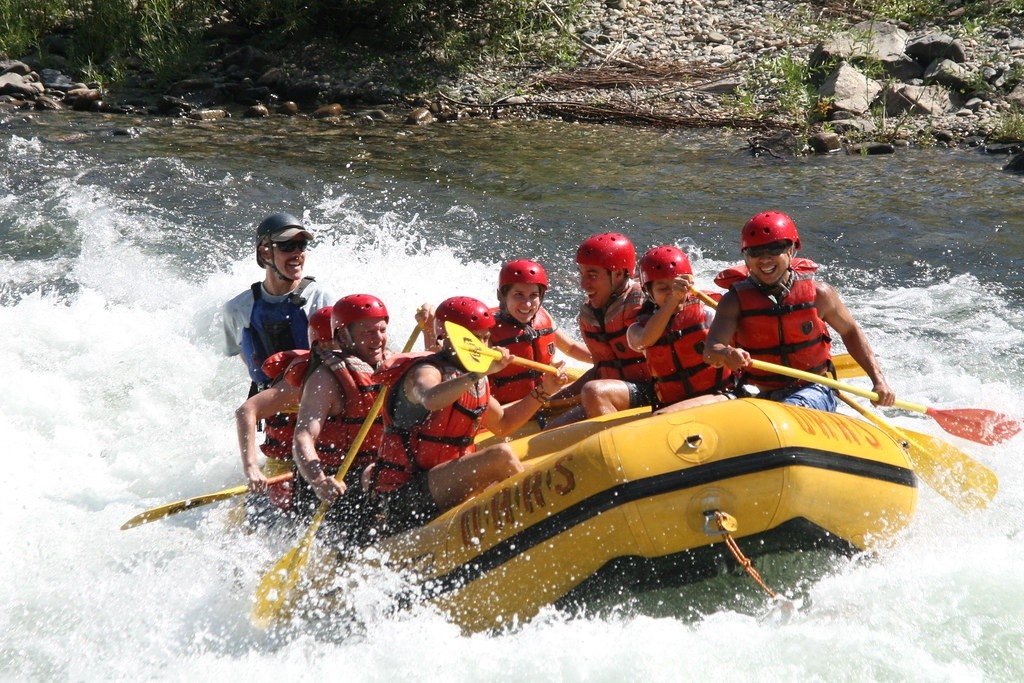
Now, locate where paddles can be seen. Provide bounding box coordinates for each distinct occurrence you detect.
[675,273,998,513]
[544,352,869,409]
[121,469,297,533]
[745,357,1024,446]
[443,321,587,382]
[250,308,428,633]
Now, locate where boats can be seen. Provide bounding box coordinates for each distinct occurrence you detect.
[223,396,918,635]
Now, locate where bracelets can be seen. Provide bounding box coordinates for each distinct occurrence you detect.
[469,371,482,384]
[530,383,551,406]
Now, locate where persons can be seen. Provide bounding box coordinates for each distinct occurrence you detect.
[702,211,895,413]
[235,232,741,552]
[221,211,338,392]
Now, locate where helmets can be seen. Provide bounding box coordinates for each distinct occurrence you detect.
[256,213,314,269]
[639,245,694,293]
[309,306,334,345]
[740,209,802,255]
[331,294,389,340]
[434,296,496,341]
[499,258,547,290]
[576,232,636,277]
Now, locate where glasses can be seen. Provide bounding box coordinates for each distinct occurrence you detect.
[265,240,308,252]
[743,242,791,256]
